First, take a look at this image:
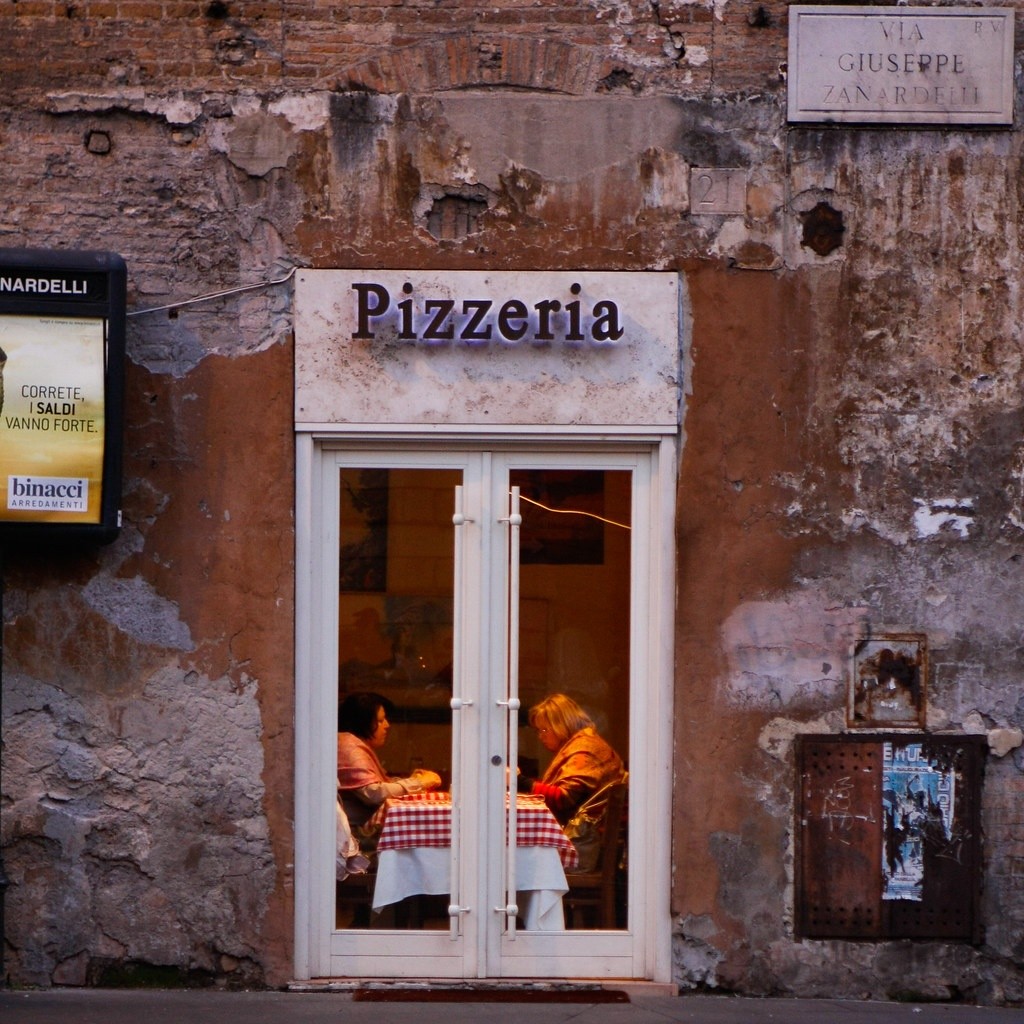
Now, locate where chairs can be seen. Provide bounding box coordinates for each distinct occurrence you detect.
[565,783,629,929]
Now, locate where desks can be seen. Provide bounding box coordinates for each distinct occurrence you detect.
[362,789,578,932]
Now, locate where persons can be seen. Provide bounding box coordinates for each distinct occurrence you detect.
[338,694,442,807]
[505,693,623,826]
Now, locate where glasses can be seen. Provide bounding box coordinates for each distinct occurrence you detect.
[535,727,554,736]
